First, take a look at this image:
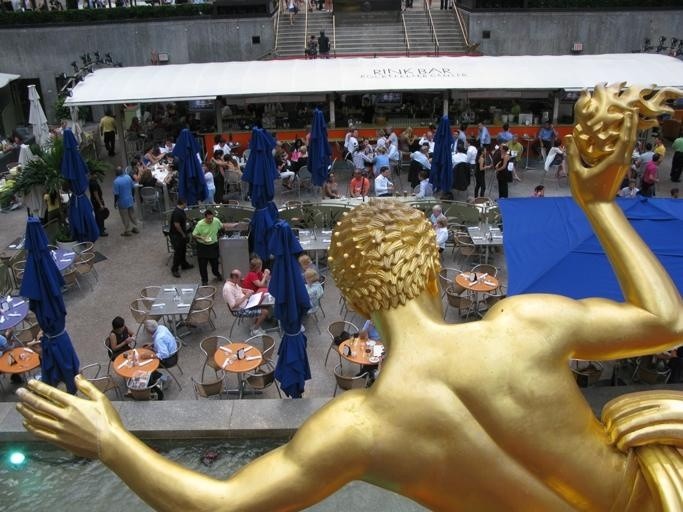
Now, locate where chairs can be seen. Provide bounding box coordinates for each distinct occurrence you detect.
[324,295,385,400]
[1,99,681,259]
[79,362,124,400]
[229,275,325,338]
[190,335,282,400]
[157,342,183,391]
[125,372,164,403]
[2,242,98,402]
[130,285,216,346]
[438,265,507,324]
[570,346,681,388]
[102,337,136,374]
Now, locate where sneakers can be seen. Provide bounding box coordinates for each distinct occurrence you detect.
[163,376,173,390]
[108,152,115,156]
[10,200,22,210]
[250,324,266,337]
[182,264,193,270]
[171,268,181,278]
[203,275,222,287]
[121,231,131,236]
[132,228,140,233]
[100,232,109,237]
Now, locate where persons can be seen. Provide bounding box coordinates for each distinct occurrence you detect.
[11,0,161,11]
[616,132,683,198]
[42,105,523,402]
[15,107,683,511]
[658,348,682,384]
[281,0,333,59]
[531,120,566,197]
[405,0,448,10]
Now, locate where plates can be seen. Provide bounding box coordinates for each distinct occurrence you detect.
[366,339,376,345]
[368,356,380,363]
